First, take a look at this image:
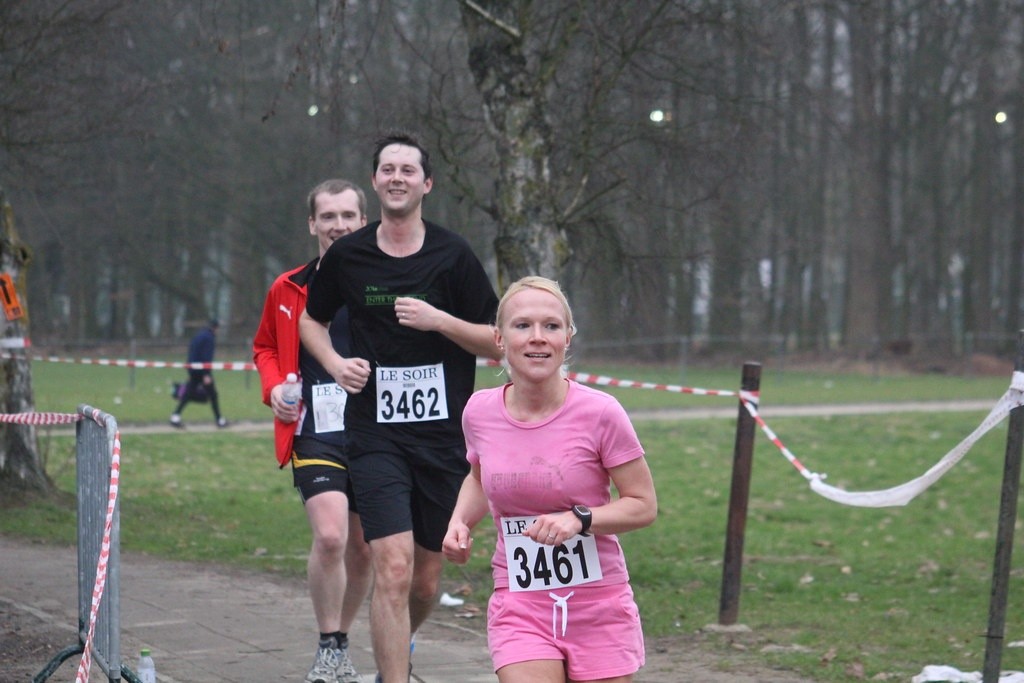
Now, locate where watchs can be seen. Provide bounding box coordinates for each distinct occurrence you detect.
[573,504,592,537]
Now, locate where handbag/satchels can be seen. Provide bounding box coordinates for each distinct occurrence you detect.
[171,381,207,402]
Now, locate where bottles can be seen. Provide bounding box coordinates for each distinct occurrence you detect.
[137,649,155,683]
[283,373,300,423]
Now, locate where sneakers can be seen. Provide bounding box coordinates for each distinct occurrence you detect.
[337,639,364,683]
[304,637,339,683]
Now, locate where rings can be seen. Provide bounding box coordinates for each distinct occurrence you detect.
[548,535,555,539]
[402,312,404,319]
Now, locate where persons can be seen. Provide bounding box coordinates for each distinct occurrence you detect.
[169,318,232,429]
[253,179,367,683]
[298,135,506,683]
[441,276,658,683]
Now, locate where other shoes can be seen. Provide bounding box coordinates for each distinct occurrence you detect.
[170,415,185,428]
[218,419,232,428]
[375,642,424,683]
